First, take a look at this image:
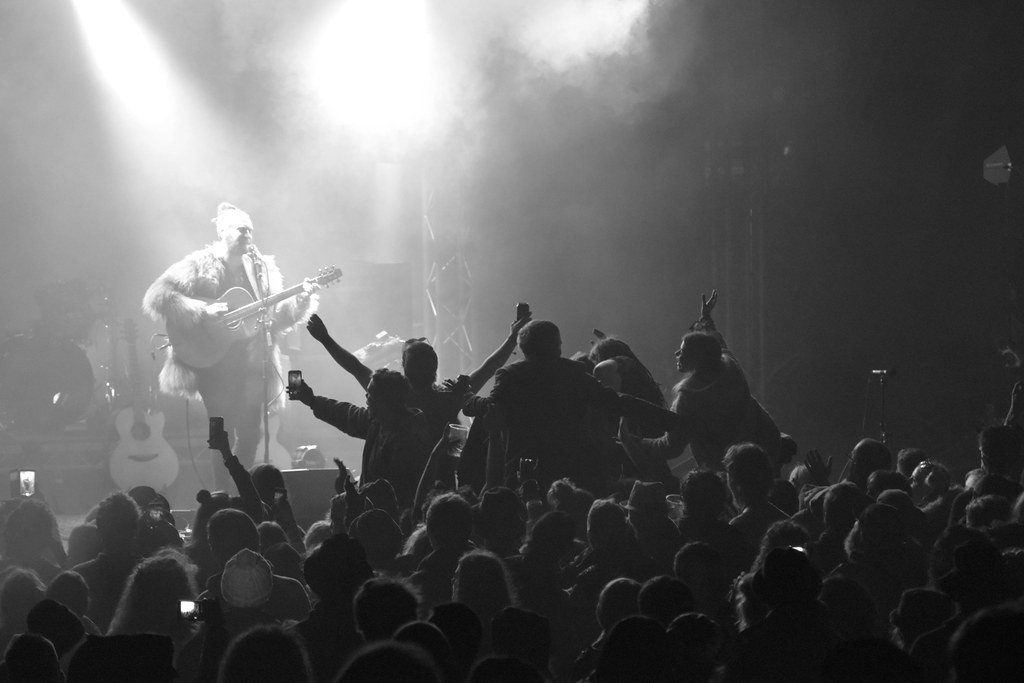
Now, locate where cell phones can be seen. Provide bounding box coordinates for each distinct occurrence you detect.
[516,302,529,321]
[288,370,302,396]
[19,470,36,499]
[209,417,224,443]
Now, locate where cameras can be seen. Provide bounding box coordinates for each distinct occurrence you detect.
[178,600,208,620]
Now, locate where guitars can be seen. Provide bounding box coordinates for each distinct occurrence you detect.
[105,317,181,496]
[161,262,344,375]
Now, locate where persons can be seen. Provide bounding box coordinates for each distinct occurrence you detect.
[142,202,321,470]
[0,291,1024,683]
[285,368,426,516]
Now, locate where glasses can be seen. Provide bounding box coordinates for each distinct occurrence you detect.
[848,453,856,463]
[403,337,431,361]
[921,458,932,475]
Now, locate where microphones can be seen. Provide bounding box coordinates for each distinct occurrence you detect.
[248,245,264,264]
[873,367,897,377]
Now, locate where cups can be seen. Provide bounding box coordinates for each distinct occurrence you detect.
[448,424,468,458]
[519,456,539,482]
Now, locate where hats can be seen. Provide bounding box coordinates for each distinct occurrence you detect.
[221,548,273,607]
[753,547,821,602]
[619,480,675,512]
[304,533,374,595]
[350,509,403,559]
[472,486,528,537]
[0,633,66,683]
[889,587,955,631]
[27,600,86,659]
[128,486,170,514]
[596,578,642,630]
